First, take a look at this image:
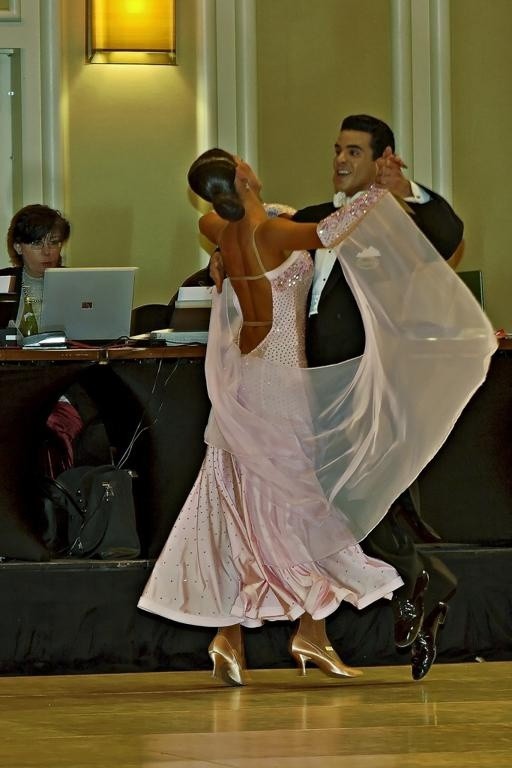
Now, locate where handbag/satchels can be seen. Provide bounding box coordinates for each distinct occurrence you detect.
[48,463,141,560]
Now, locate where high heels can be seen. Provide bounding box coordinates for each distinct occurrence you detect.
[207,634,246,686]
[292,634,363,678]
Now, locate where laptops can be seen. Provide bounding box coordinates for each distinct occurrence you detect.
[39,267,136,345]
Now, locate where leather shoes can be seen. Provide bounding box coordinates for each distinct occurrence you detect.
[410,601,448,680]
[394,571,431,648]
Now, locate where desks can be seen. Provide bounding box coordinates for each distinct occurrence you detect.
[1,337,512,561]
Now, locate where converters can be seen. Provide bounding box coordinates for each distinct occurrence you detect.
[128,340,165,348]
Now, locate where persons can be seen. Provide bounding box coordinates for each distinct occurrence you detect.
[0,201,108,444]
[134,146,501,688]
[157,201,299,329]
[209,109,468,681]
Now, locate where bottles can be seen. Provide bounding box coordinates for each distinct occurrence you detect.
[17,297,38,338]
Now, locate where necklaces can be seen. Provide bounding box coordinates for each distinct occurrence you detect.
[20,282,43,305]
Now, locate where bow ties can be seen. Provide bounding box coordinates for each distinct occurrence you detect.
[333,191,362,208]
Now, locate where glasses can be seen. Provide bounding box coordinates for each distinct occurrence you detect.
[30,240,60,251]
